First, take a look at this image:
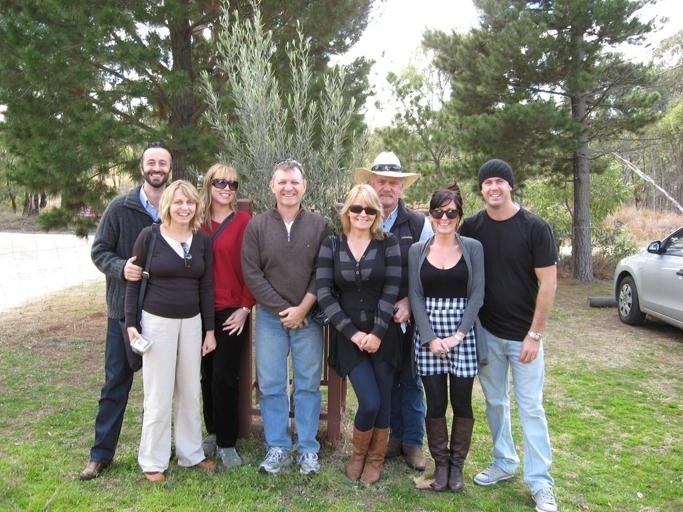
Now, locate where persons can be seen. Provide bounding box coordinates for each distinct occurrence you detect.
[77,142,173,480]
[242,160,335,475]
[193,164,256,467]
[317,184,401,481]
[353,151,435,472]
[457,159,558,512]
[409,188,484,492]
[124,179,218,482]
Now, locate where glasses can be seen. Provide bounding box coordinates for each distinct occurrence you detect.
[181,242,193,269]
[209,179,239,191]
[429,209,460,219]
[349,205,378,215]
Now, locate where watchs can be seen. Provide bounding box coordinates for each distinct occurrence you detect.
[242,305,250,312]
[528,329,546,340]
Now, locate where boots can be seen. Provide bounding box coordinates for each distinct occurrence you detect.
[360,427,392,485]
[345,423,375,480]
[448,416,475,493]
[424,417,448,492]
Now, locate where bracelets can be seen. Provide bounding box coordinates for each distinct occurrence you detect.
[454,334,462,340]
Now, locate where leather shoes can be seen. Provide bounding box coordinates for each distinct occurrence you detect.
[143,472,164,482]
[402,447,427,470]
[385,441,402,457]
[196,458,216,471]
[81,460,103,480]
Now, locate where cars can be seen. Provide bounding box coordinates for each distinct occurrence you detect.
[613,225,682,330]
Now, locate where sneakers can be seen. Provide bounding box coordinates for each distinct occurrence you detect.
[296,451,319,475]
[473,463,515,485]
[215,446,242,469]
[202,434,218,456]
[530,488,558,512]
[257,447,293,475]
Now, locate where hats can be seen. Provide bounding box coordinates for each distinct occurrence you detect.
[478,159,513,189]
[354,151,421,193]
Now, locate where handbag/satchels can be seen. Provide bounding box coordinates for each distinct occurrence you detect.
[118,223,157,371]
